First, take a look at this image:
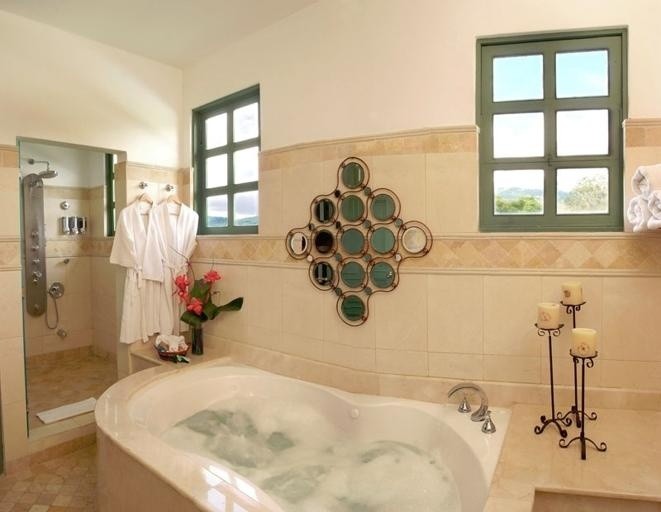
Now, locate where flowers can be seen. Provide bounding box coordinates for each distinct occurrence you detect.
[161,246,245,327]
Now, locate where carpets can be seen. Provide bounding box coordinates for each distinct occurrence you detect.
[37,396,98,424]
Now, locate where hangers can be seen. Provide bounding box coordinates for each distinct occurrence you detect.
[159,184,182,215]
[131,182,152,214]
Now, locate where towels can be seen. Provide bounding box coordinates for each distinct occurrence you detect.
[646,191,661,228]
[627,197,650,233]
[632,163,661,194]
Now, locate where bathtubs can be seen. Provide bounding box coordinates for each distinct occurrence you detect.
[90,355,510,512]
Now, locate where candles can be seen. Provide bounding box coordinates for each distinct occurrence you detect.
[538,302,560,331]
[571,328,598,356]
[564,284,582,305]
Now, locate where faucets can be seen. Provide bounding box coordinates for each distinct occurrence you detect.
[447,382,489,422]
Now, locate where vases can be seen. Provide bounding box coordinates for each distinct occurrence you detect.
[190,325,204,354]
